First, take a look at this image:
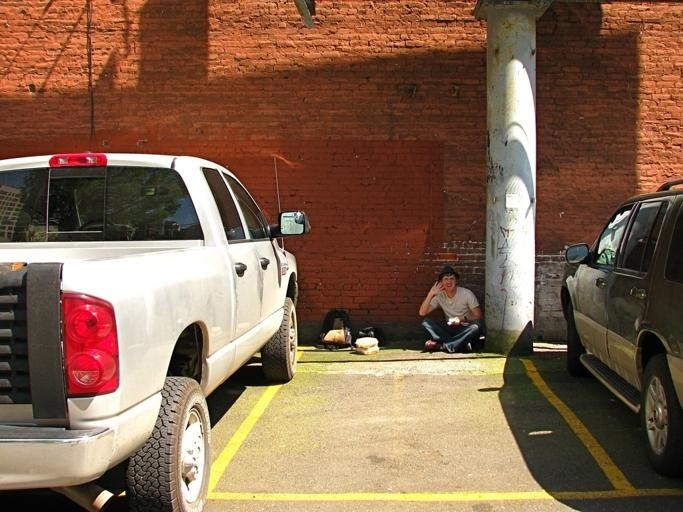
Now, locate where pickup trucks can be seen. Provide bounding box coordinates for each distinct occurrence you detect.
[1,152,311,508]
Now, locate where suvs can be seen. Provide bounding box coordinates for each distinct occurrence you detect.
[561,177,683,476]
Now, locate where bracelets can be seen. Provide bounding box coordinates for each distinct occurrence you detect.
[464,315,467,323]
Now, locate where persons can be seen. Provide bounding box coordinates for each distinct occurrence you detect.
[418,265,482,353]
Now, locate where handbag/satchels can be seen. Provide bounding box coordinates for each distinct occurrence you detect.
[353,327,384,346]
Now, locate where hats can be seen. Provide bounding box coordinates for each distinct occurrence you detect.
[439,266,459,279]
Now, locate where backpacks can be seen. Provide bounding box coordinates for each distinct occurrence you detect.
[320,310,352,349]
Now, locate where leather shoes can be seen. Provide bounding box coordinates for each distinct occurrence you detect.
[462,342,472,352]
[424,340,437,351]
